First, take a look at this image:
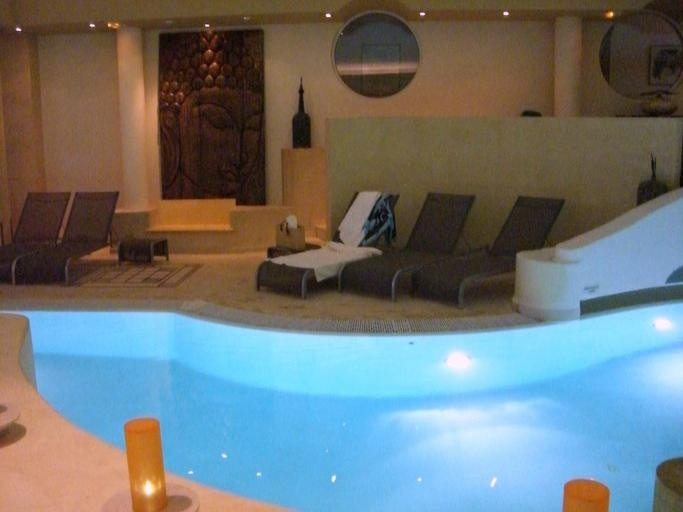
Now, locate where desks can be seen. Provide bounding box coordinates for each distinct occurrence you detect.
[117,235,169,265]
[264,243,320,259]
[280,147,328,233]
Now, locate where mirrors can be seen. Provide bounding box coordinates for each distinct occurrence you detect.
[331,10,421,99]
[596,9,682,101]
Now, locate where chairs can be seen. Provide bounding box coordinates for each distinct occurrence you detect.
[337,192,477,300]
[410,195,566,308]
[11,189,119,282]
[254,191,404,299]
[0,190,72,285]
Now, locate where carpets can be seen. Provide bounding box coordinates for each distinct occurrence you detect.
[56,259,204,287]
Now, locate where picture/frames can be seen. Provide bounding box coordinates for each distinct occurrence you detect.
[359,42,400,96]
[644,45,680,87]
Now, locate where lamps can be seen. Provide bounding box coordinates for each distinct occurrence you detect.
[125,416,169,512]
